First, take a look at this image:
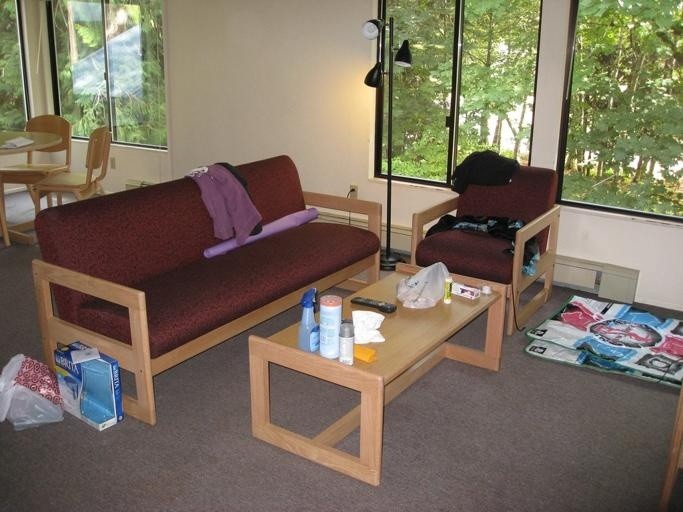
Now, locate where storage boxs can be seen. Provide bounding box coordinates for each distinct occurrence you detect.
[53,339,123,432]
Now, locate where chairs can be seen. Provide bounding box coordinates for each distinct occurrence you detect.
[0,115,72,229]
[31,124,115,226]
[409,160,563,340]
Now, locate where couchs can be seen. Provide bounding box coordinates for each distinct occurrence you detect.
[29,152,385,433]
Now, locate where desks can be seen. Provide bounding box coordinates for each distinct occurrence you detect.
[242,260,510,488]
[0,128,63,249]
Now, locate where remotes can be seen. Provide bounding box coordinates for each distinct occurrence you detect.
[350,297,397,314]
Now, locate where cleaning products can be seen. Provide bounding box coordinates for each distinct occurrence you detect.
[298,287,320,352]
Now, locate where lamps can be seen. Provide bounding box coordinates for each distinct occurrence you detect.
[360,15,413,271]
